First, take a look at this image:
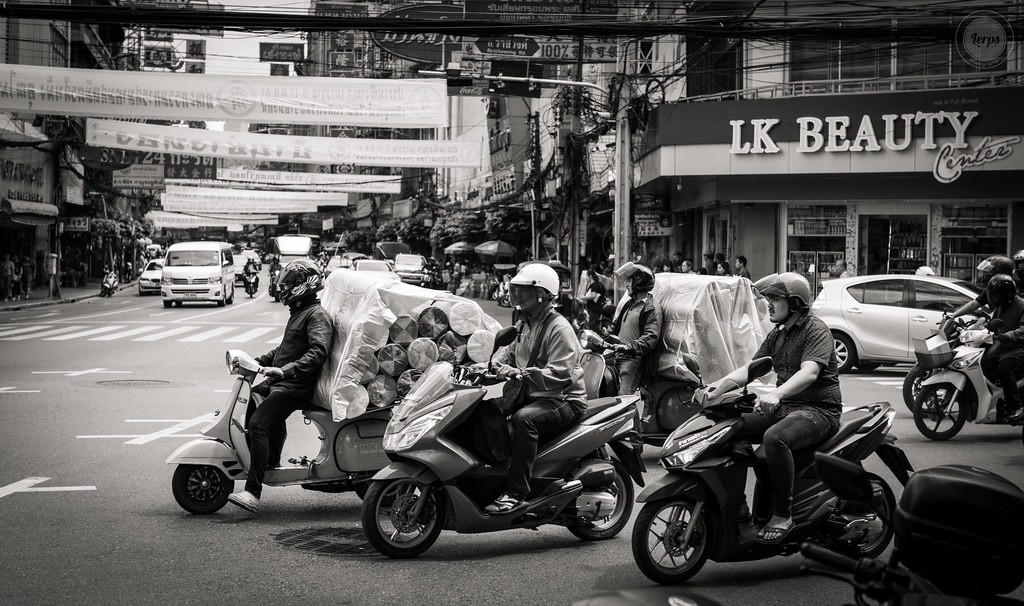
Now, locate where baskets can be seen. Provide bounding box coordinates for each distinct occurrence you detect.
[914,348,960,369]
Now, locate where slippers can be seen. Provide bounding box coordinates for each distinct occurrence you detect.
[755,521,797,544]
[486,491,530,516]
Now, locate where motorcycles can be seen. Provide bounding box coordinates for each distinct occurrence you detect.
[903,302,1024,441]
[516,259,573,324]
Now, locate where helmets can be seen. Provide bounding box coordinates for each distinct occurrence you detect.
[915,265,935,278]
[985,272,1017,308]
[273,256,280,263]
[276,258,326,306]
[507,263,560,296]
[976,255,1017,287]
[1011,249,1024,276]
[248,257,256,263]
[622,262,656,295]
[759,271,811,310]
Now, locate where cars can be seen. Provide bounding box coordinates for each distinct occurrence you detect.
[394,252,428,284]
[811,273,993,375]
[322,252,401,282]
[233,250,262,287]
[137,259,164,297]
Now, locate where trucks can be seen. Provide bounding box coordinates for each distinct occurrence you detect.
[274,236,312,270]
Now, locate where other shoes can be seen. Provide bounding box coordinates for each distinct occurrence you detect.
[227,491,261,514]
[1008,401,1024,423]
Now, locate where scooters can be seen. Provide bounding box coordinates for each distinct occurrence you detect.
[102,270,119,298]
[164,349,416,519]
[491,273,514,308]
[571,302,616,339]
[630,353,918,588]
[361,326,648,562]
[571,462,1024,606]
[241,270,259,298]
[420,266,438,289]
[268,270,280,302]
[575,327,704,447]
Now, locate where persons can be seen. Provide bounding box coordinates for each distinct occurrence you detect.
[422,256,436,271]
[660,251,751,280]
[228,258,334,512]
[604,262,664,395]
[693,272,843,545]
[0,252,36,302]
[949,249,1024,416]
[482,263,588,513]
[579,270,604,334]
[243,257,259,292]
[835,259,851,278]
[446,256,468,295]
[269,256,282,277]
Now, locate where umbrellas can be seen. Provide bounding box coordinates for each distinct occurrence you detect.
[444,241,478,254]
[475,240,517,264]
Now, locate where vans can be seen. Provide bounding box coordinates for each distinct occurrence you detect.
[161,241,234,308]
